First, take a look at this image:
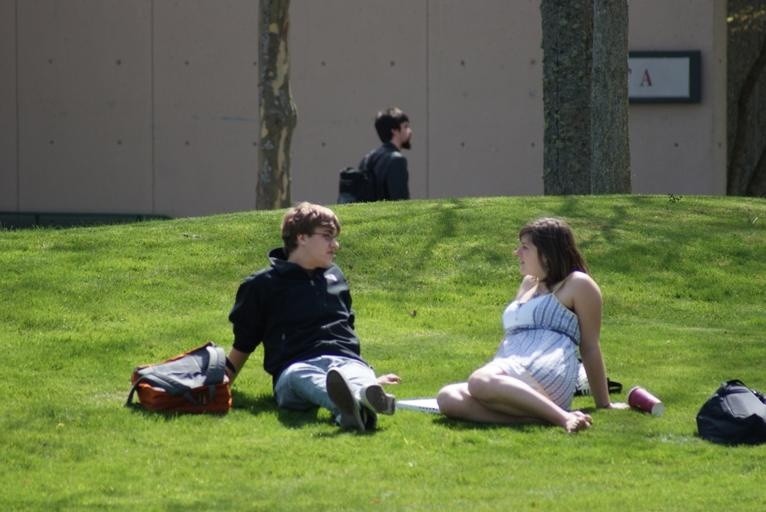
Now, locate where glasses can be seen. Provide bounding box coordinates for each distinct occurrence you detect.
[311,232,334,240]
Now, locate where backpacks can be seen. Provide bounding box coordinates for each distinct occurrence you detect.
[124,340,232,412]
[696,379,766,444]
[337,149,385,204]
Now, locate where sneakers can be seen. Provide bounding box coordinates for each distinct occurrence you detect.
[326,367,396,433]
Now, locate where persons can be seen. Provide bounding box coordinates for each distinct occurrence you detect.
[230,200,402,432]
[436,217,631,434]
[358,107,414,199]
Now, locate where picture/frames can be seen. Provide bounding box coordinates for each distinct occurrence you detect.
[628,51,700,104]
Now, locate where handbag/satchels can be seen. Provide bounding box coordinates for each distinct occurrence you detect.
[573,359,622,397]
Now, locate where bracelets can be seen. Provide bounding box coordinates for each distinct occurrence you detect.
[604,402,611,408]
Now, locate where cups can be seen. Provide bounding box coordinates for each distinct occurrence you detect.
[627,385,664,416]
